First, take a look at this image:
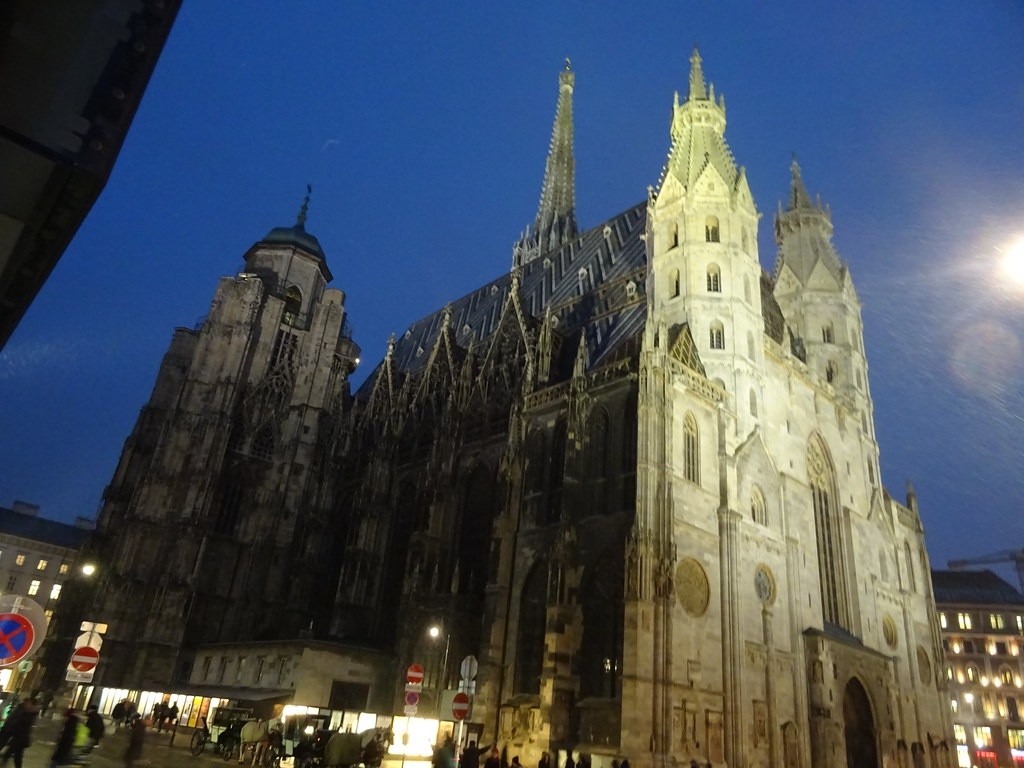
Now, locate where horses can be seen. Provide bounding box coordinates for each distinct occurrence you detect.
[325,724,395,768]
[238,717,282,768]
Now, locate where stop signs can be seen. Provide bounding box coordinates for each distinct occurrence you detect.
[451,693,470,721]
[406,663,424,684]
[71,646,100,673]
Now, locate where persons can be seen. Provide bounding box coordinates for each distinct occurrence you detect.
[0,677,717,768]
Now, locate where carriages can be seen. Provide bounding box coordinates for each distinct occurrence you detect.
[263,712,395,768]
[189,706,285,767]
